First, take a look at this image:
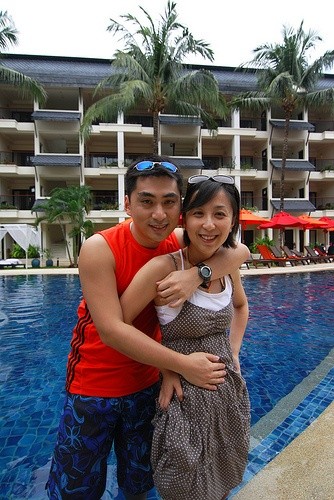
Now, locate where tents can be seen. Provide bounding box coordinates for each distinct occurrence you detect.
[0,224,41,269]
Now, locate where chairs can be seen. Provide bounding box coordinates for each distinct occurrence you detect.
[240,245,334,270]
[0,259,26,269]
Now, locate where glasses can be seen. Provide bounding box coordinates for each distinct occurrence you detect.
[131,160,177,174]
[188,174,235,185]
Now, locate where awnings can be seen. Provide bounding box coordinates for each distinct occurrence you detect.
[170,158,205,174]
[32,155,83,183]
[31,111,82,137]
[158,115,203,142]
[269,160,316,185]
[269,120,315,145]
[270,200,316,219]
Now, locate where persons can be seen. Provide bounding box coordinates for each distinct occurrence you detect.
[45,162,250,500]
[120,174,251,500]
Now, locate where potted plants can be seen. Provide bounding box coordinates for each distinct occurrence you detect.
[30,245,41,268]
[44,250,54,266]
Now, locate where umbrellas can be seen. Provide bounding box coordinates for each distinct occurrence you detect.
[238,208,334,247]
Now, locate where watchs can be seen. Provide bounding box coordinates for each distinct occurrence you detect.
[196,262,212,281]
